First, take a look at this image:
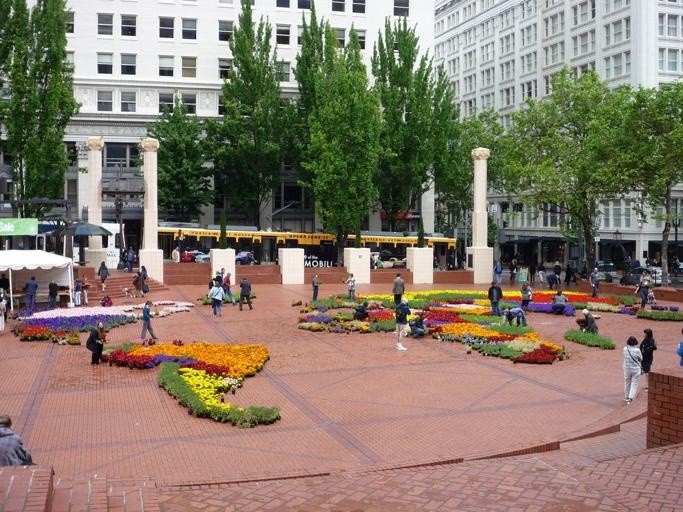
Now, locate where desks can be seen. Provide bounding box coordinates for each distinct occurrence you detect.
[7,293,25,311]
[15,290,68,307]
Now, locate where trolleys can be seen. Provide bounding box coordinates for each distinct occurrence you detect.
[122,275,143,299]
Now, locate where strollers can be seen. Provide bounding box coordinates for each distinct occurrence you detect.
[646,289,656,304]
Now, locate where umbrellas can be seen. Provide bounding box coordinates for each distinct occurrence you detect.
[59,221,113,262]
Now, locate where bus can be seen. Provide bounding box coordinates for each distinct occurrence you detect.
[250,231,365,271]
[157,227,291,266]
[10,219,129,269]
[342,233,458,272]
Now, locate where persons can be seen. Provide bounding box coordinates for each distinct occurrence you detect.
[433,257,438,271]
[639,329,657,389]
[346,273,356,299]
[635,270,654,309]
[392,273,404,305]
[589,268,601,298]
[354,302,369,321]
[248,251,259,266]
[0,414,37,468]
[1,246,157,365]
[581,309,599,336]
[172,247,181,263]
[208,268,253,317]
[312,274,319,301]
[370,256,384,269]
[489,259,572,326]
[181,247,192,263]
[622,336,643,404]
[395,300,411,351]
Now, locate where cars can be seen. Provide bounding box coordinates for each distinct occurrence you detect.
[183,250,258,266]
[571,257,617,282]
[620,265,672,288]
[370,252,407,269]
